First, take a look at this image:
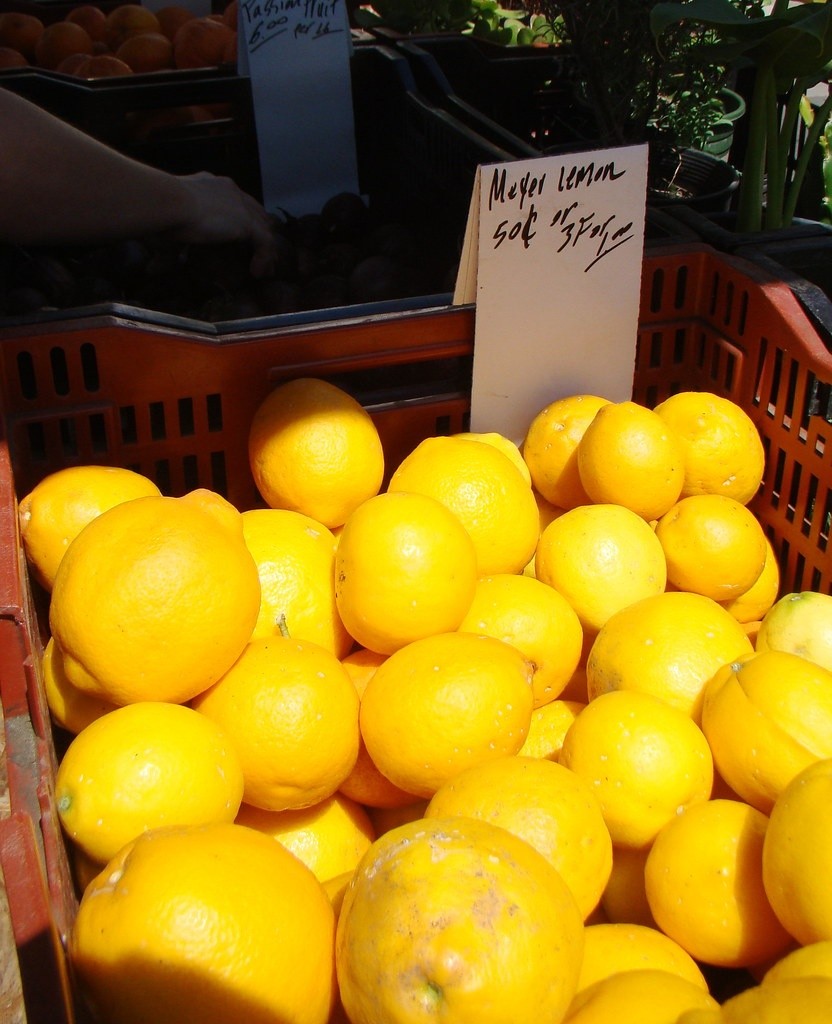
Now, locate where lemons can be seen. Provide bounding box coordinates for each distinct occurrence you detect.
[16,379,832,1024]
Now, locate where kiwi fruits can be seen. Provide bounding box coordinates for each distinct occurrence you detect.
[4,192,444,321]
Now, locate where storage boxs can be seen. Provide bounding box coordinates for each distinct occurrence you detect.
[0,0,832,1023]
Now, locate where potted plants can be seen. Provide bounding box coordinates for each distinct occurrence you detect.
[604,0,744,164]
[514,0,735,224]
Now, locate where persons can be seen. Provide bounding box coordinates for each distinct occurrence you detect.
[0,88,279,281]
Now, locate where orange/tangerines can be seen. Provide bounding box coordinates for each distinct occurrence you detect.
[0,0,236,121]
